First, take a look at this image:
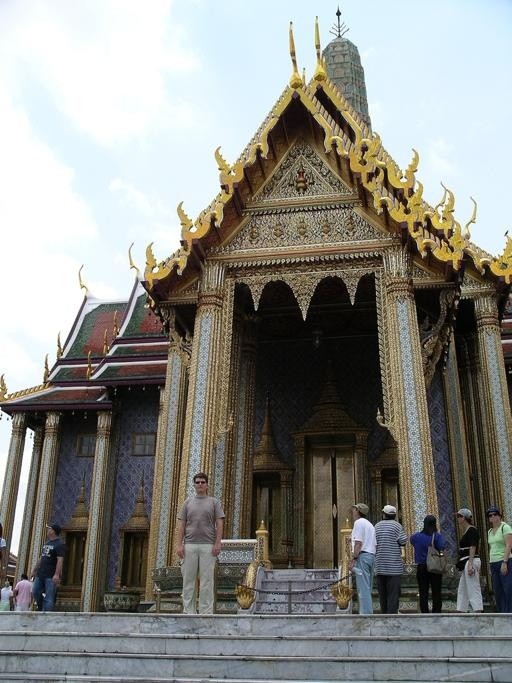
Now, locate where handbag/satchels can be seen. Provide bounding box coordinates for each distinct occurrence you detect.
[427,546,447,574]
[455,560,465,571]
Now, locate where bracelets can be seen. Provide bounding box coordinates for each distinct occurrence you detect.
[352,556,358,560]
[502,558,509,562]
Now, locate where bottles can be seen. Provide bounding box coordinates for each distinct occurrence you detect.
[350,567,363,575]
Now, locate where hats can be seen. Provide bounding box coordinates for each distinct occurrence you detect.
[352,503,369,516]
[382,504,396,514]
[486,507,500,513]
[452,509,473,519]
[46,522,61,533]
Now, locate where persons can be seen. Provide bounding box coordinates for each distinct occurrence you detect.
[345,502,377,613]
[1,580,14,611]
[410,513,447,614]
[176,471,226,614]
[452,507,486,613]
[374,503,409,614]
[486,507,512,613]
[12,572,33,611]
[29,522,66,611]
[0,522,7,580]
